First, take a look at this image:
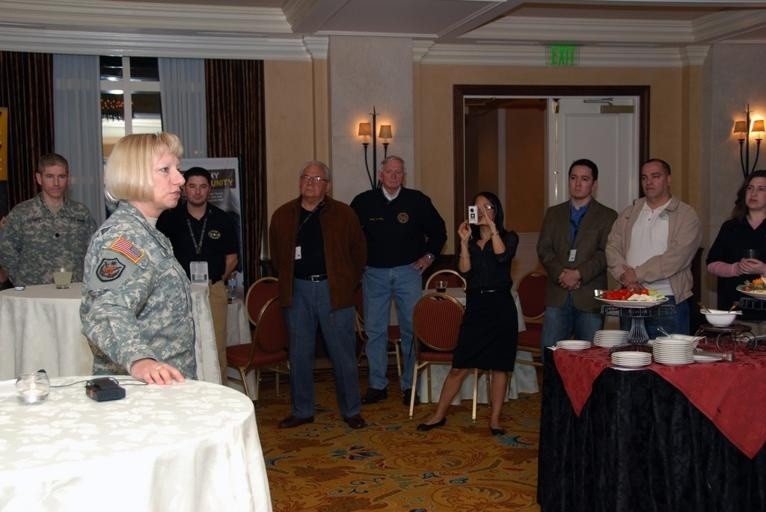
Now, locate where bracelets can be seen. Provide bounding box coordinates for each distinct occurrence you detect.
[459,254,470,259]
[490,232,499,237]
[425,253,435,261]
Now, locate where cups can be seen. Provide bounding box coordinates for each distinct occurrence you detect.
[51,267,72,288]
[436,280,446,295]
[16,372,49,405]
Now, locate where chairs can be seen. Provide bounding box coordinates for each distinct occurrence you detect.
[222,264,550,424]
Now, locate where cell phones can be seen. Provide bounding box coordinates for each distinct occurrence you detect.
[469,206,478,224]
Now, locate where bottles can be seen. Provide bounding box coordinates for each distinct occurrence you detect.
[229,275,238,296]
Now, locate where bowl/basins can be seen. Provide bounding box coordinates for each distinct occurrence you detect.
[701,311,738,329]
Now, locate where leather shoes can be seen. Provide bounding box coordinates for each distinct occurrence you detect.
[488,423,505,435]
[342,413,366,429]
[401,387,419,406]
[279,414,314,428]
[359,387,388,404]
[415,417,447,432]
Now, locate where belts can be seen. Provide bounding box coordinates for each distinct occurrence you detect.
[365,262,395,267]
[294,273,327,282]
[475,288,500,293]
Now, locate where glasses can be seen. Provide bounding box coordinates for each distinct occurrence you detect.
[300,174,328,182]
[483,204,494,209]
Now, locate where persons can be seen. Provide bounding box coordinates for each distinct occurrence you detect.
[704,170,766,324]
[604,159,701,338]
[78,132,198,386]
[1,214,14,290]
[155,166,240,286]
[269,161,369,428]
[536,159,618,361]
[0,152,98,288]
[416,190,520,434]
[347,156,448,407]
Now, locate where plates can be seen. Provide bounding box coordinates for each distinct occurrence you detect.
[736,285,766,298]
[612,351,651,368]
[594,296,669,307]
[593,329,631,347]
[653,337,696,365]
[558,340,592,351]
[693,356,722,363]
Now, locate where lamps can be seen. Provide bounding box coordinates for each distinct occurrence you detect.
[730,117,765,179]
[356,122,393,191]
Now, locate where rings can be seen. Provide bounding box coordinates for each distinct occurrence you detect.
[156,366,161,370]
